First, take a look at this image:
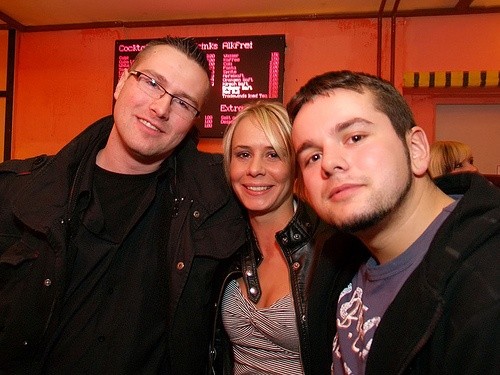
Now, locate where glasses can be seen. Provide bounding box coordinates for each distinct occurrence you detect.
[128,68,202,121]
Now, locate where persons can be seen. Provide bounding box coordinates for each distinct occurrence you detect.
[208,101,337,375]
[0,35,251,375]
[287,70,500,375]
[427,140,480,179]
